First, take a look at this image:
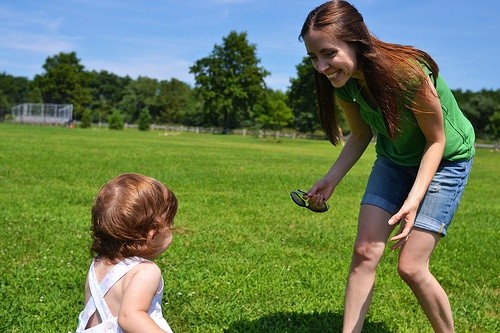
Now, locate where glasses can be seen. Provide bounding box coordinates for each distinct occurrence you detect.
[290,189,330,212]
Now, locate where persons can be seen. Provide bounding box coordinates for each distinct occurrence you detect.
[67,170,179,333]
[296,0,476,333]
